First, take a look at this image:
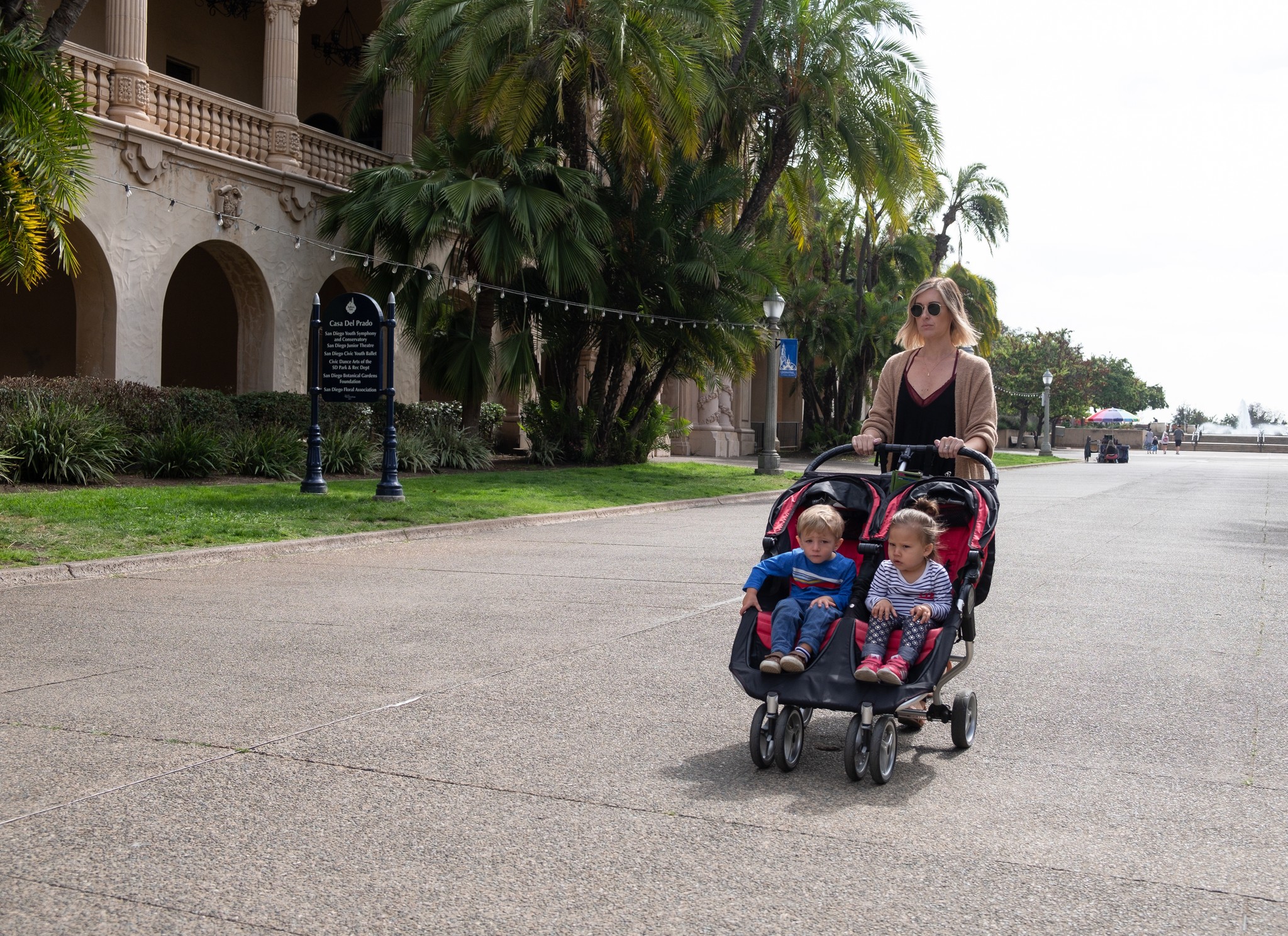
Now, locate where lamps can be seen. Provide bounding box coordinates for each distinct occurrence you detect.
[195,0,265,19]
[311,0,371,68]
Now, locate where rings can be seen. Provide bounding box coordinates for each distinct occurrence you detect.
[944,444,950,450]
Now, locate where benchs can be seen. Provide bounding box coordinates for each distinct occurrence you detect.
[1010,436,1027,448]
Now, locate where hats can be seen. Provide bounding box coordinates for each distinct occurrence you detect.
[1177,424,1181,427]
[1164,432,1169,435]
[1154,436,1157,439]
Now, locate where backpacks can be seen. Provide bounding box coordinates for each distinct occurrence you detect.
[1163,436,1168,442]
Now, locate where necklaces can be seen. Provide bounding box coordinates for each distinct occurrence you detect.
[922,346,956,376]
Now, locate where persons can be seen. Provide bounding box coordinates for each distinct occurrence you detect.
[1166,424,1170,435]
[1153,417,1158,422]
[1105,440,1119,463]
[1144,428,1153,454]
[1150,435,1159,454]
[854,498,952,685]
[1030,430,1037,448]
[1147,423,1151,431]
[851,277,998,731]
[1161,431,1169,454]
[1173,426,1185,454]
[740,504,857,674]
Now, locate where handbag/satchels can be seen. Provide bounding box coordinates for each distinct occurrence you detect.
[1144,440,1148,445]
[1172,435,1176,442]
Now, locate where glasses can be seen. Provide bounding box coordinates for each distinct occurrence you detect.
[910,303,947,318]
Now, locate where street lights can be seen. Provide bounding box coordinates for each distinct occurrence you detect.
[1037,367,1054,457]
[752,281,786,476]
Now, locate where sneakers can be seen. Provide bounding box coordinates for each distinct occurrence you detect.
[877,654,910,685]
[854,653,883,683]
[759,652,784,673]
[780,651,807,672]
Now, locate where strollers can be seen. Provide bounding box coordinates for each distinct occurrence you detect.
[730,439,1000,782]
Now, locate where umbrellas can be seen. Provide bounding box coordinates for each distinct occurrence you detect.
[1084,406,1140,442]
[1052,415,1087,428]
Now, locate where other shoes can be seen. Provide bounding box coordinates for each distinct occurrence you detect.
[1164,451,1166,454]
[1106,460,1109,463]
[1116,462,1119,463]
[1153,453,1154,454]
[1150,452,1151,454]
[1176,452,1179,454]
[898,704,927,728]
[1147,453,1149,454]
[1156,453,1157,454]
